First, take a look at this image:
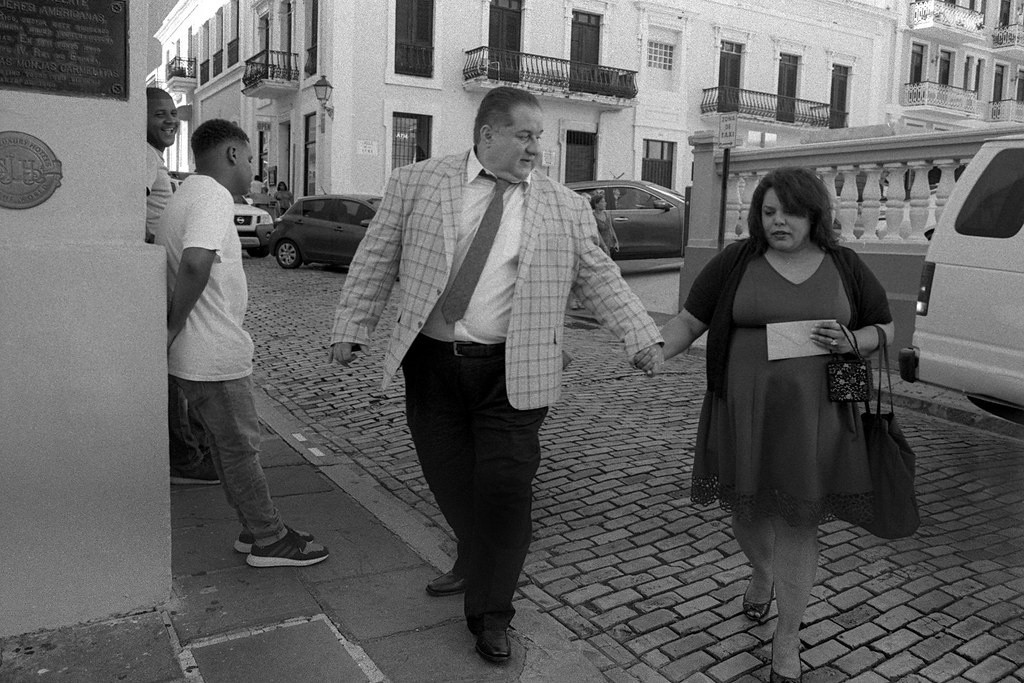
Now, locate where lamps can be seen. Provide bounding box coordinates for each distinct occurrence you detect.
[313,75,334,119]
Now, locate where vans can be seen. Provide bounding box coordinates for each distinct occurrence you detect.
[897,133,1024,426]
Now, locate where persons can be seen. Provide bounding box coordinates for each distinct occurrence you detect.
[591,194,619,258]
[569,290,586,309]
[249,174,265,193]
[145,86,221,485]
[326,88,665,668]
[633,166,896,683]
[153,118,331,568]
[274,182,294,216]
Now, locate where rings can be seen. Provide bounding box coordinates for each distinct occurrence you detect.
[831,340,839,346]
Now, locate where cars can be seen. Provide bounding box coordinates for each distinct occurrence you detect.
[168,171,275,258]
[562,179,685,261]
[269,195,382,270]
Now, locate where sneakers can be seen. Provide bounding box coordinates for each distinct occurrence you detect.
[234,523,314,553]
[245,527,329,567]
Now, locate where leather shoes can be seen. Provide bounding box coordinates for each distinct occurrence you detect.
[467,623,512,662]
[425,571,466,596]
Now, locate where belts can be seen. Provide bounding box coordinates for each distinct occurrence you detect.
[413,334,505,358]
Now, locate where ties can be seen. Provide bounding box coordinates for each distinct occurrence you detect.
[441,179,512,324]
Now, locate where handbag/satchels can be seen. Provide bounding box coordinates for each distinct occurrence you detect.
[832,326,920,539]
[825,322,874,402]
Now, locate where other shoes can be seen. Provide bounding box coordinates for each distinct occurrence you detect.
[170,457,221,484]
[770,629,802,683]
[743,573,775,619]
